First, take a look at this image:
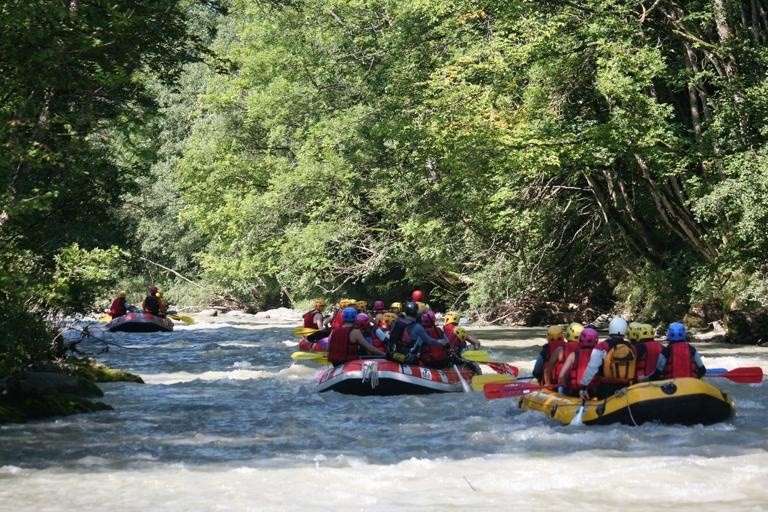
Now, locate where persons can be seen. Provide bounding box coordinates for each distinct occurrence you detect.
[299,289,483,376]
[532,314,707,401]
[107,290,139,320]
[141,286,170,317]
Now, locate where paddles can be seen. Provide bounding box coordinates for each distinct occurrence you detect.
[460,350,492,362]
[472,374,534,392]
[484,379,563,398]
[703,367,763,383]
[166,313,194,325]
[290,351,386,360]
[482,362,519,377]
[293,326,325,336]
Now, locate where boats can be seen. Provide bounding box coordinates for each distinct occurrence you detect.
[298,336,330,353]
[517,377,736,427]
[104,312,174,332]
[315,359,518,397]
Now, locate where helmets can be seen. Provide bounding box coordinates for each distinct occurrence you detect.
[666,322,686,341]
[411,290,422,300]
[608,317,654,341]
[546,322,598,347]
[119,291,125,297]
[315,300,325,311]
[151,287,163,299]
[445,313,467,342]
[341,301,435,326]
